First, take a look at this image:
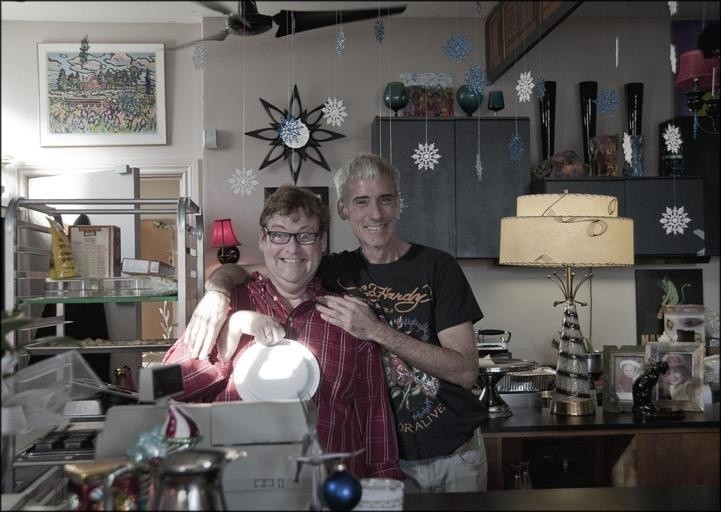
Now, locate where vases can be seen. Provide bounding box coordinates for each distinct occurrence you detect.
[536,81,646,176]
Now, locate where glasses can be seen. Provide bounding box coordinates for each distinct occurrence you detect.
[263,226,320,245]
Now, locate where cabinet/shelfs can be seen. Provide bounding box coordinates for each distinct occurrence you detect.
[544,176,706,260]
[1,198,203,419]
[483,429,721,490]
[371,117,531,264]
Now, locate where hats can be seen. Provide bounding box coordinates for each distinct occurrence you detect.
[662,353,685,366]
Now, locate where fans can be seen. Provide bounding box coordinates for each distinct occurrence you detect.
[166,1,408,53]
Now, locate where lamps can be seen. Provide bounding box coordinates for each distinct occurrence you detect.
[498,187,634,420]
[674,50,721,140]
[209,218,242,264]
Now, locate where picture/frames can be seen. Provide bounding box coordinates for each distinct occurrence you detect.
[37,42,168,147]
[604,342,706,414]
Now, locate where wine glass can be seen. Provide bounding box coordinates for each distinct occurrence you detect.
[488,90,504,116]
[383,82,409,117]
[458,84,482,118]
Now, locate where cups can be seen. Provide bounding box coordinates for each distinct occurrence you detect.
[355,476,405,511]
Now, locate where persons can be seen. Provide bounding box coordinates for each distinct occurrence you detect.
[162,185,406,485]
[660,353,693,404]
[620,354,639,394]
[182,152,486,492]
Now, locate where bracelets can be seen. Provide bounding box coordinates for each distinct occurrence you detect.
[207,289,232,301]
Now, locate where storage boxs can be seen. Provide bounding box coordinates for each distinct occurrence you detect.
[67,225,121,296]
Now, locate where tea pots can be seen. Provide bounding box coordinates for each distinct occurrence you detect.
[144,446,246,511]
